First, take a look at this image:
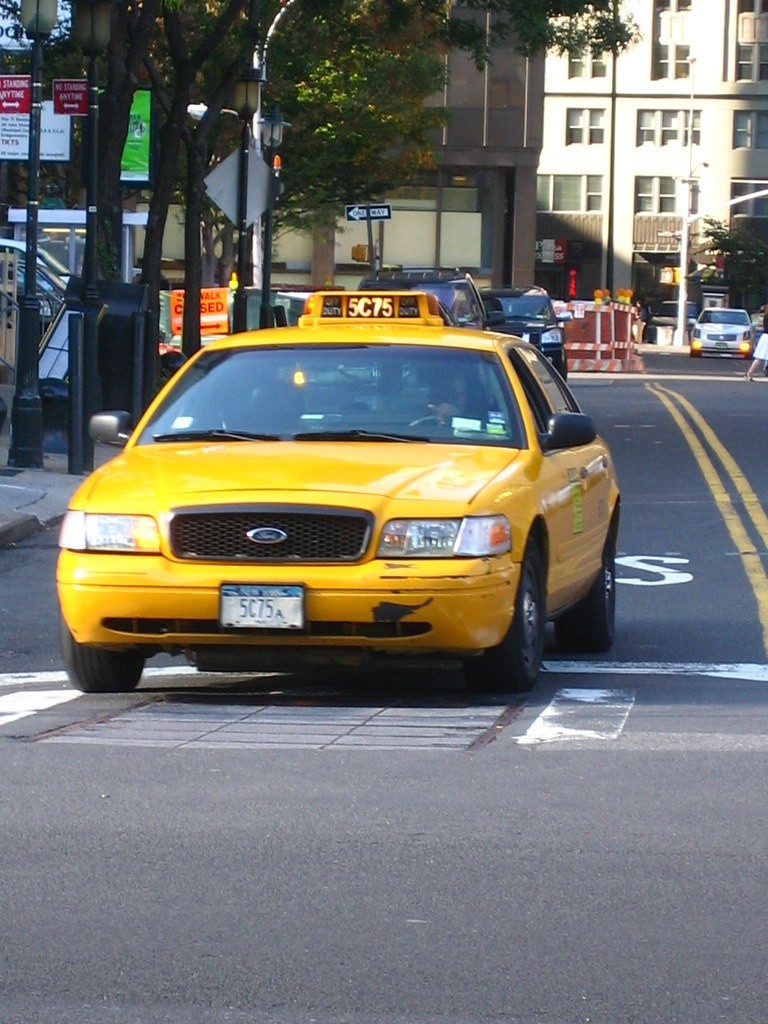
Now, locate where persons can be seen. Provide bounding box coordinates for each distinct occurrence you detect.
[635,301,649,341]
[747,313,768,382]
[427,371,486,429]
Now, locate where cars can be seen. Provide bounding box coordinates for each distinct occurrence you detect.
[0,239,74,333]
[688,306,759,358]
[51,289,625,696]
[647,300,703,336]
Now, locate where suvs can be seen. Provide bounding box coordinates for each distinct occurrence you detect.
[357,264,506,331]
[477,284,573,383]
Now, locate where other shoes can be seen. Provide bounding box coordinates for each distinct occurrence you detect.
[744,374,754,383]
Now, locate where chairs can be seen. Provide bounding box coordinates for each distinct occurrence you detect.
[254,382,306,424]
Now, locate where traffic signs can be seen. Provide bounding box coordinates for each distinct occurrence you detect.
[344,203,392,222]
[657,230,681,237]
[0,75,30,114]
[52,78,89,117]
[170,288,230,338]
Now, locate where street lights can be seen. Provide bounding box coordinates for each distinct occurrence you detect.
[256,102,295,330]
[673,55,712,346]
[6,0,58,468]
[186,0,300,339]
